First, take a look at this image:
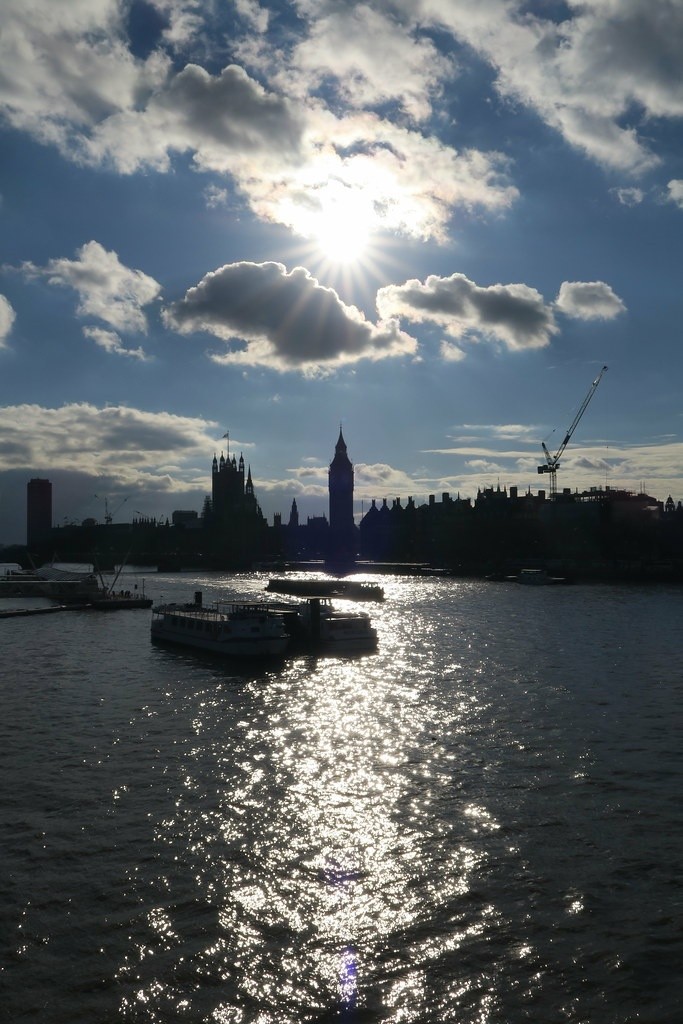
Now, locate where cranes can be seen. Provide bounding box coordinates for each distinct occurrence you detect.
[103,495,131,524]
[535,364,610,506]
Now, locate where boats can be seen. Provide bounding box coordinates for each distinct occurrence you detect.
[150,588,300,660]
[485,572,518,581]
[267,575,386,601]
[290,597,379,654]
[410,565,454,578]
[515,568,568,586]
